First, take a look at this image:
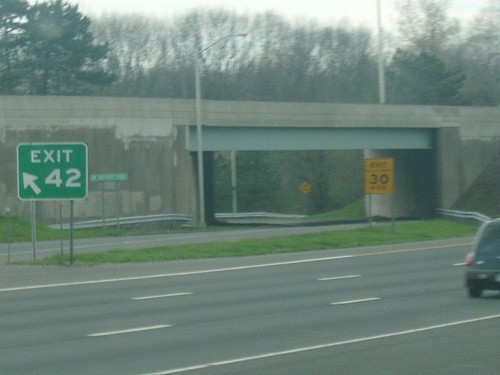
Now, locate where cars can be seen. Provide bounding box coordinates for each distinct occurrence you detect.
[465,218,500,298]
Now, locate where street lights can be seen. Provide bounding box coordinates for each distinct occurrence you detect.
[191,32,249,230]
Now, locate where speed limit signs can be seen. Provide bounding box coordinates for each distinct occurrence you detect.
[364,157,394,196]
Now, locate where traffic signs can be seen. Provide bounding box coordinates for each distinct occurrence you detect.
[17,141,90,203]
